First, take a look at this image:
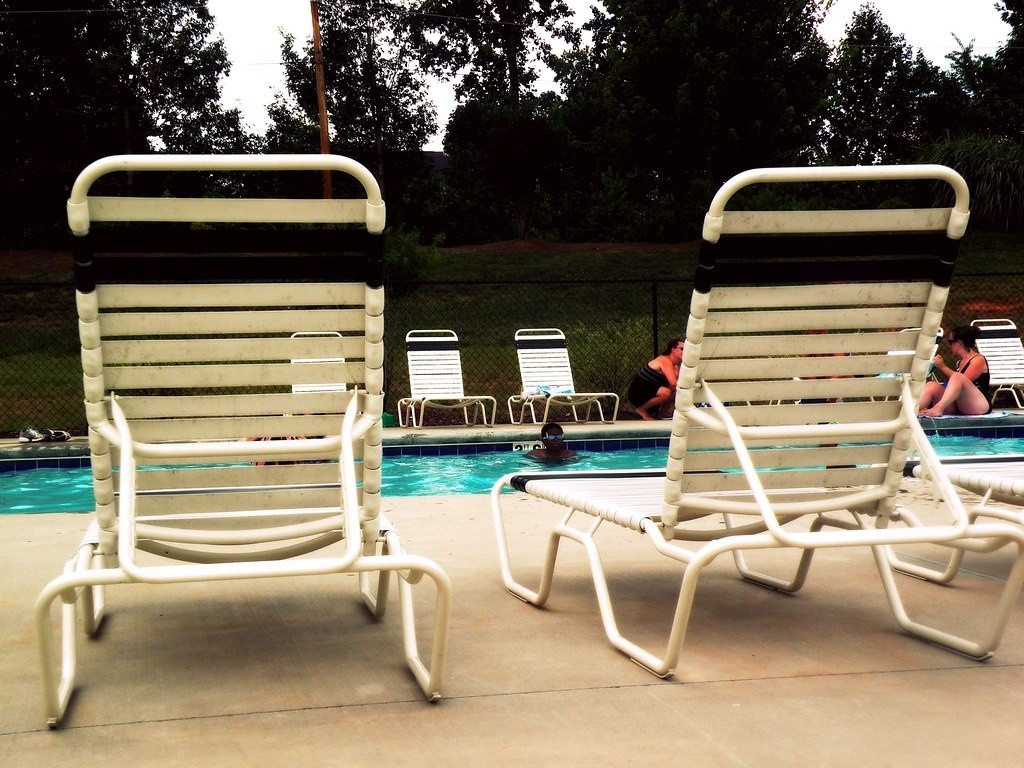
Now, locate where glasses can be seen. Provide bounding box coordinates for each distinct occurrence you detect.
[675,346,683,351]
[541,432,564,441]
[948,338,958,345]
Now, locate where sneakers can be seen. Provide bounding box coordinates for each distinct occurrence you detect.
[19,428,46,443]
[39,428,71,442]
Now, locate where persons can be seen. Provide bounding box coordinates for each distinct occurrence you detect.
[919,326,992,416]
[245,412,330,465]
[627,339,685,421]
[796,326,856,448]
[522,423,576,460]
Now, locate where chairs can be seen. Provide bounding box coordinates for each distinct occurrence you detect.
[971,317,1023,411]
[284,330,362,416]
[491,160,1024,677]
[507,329,620,424]
[38,148,450,738]
[869,326,944,403]
[397,329,496,428]
